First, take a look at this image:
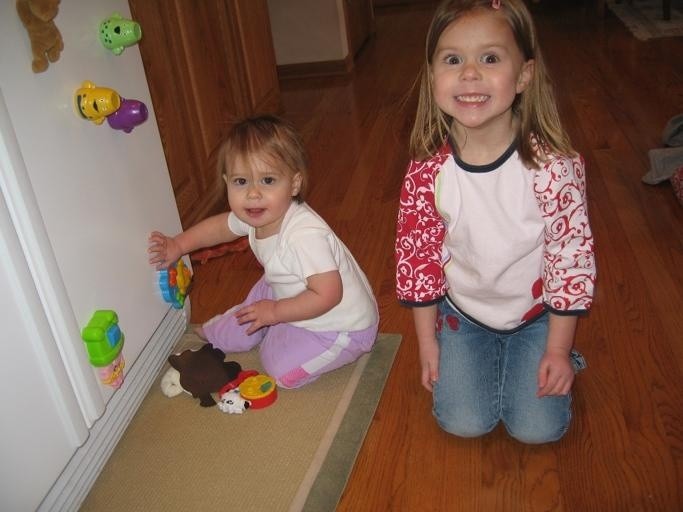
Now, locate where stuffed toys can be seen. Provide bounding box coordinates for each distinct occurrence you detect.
[14,1,64,74]
[158,342,278,417]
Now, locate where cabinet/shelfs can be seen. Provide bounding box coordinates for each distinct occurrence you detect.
[129,0,283,245]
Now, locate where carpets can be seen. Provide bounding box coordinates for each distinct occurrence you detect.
[77,319,405,511]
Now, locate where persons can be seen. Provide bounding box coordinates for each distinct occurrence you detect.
[148,112,381,390]
[393,1,600,448]
